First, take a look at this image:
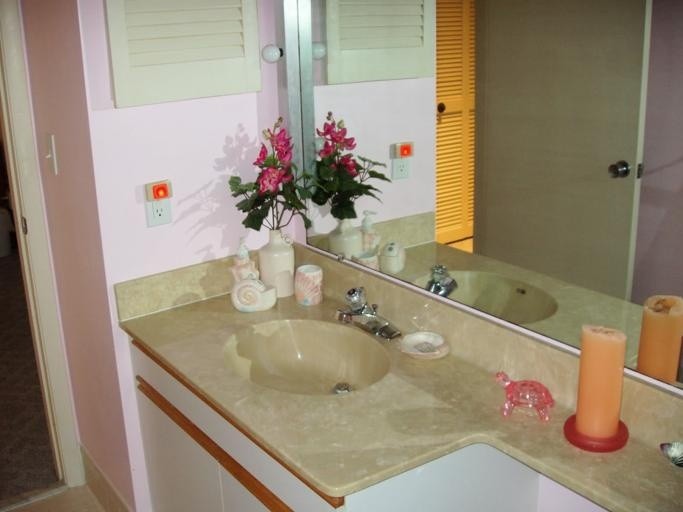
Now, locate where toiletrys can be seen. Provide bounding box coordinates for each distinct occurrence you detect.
[231,237,278,312]
[361,209,379,252]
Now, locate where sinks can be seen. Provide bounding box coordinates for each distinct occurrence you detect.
[222,315,396,398]
[411,268,560,328]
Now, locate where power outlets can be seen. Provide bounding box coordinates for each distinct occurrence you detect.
[392,159,408,181]
[145,200,171,228]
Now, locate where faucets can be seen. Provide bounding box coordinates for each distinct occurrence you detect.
[334,287,402,341]
[427,263,457,297]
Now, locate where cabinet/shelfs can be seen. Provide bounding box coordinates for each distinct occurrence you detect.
[133,388,273,511]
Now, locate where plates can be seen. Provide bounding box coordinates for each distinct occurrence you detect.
[401,332,452,358]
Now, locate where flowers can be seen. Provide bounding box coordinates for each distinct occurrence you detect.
[307,110,393,220]
[228,114,313,232]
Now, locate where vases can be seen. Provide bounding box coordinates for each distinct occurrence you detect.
[327,217,363,259]
[258,228,296,299]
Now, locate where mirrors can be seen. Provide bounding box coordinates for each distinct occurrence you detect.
[275,0,682,393]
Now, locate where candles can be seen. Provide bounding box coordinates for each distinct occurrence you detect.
[562,323,629,454]
[634,293,682,386]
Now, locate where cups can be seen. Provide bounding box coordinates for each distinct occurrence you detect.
[294,265,323,306]
[352,253,379,272]
